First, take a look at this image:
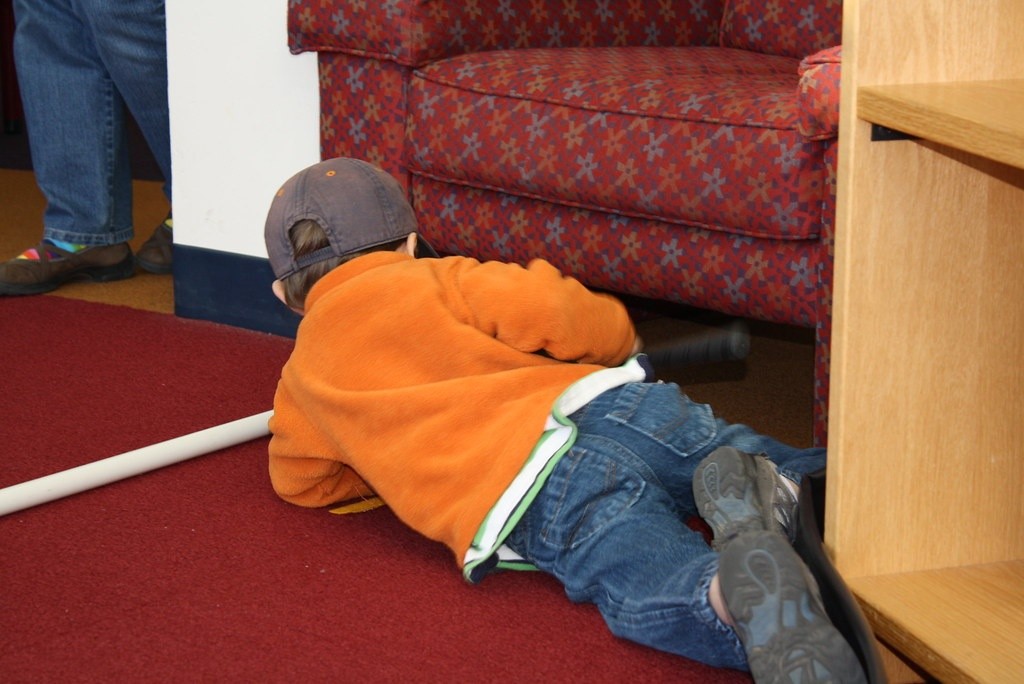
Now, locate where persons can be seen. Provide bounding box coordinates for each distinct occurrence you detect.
[1,0,173,298]
[263,158,876,684]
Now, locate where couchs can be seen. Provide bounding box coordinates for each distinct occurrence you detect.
[286,0,845,450]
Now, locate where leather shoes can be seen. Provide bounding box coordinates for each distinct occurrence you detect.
[136,218,173,275]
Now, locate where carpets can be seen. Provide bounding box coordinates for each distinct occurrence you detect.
[0,292,755,684]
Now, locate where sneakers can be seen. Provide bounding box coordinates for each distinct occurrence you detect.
[719,530,868,684]
[693,446,798,548]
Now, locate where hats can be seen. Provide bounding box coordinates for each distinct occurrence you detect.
[264,157,440,281]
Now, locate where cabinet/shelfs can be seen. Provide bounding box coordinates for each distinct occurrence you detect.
[825,0,1024,684]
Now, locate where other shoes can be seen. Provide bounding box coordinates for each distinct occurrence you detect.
[0,242,138,295]
